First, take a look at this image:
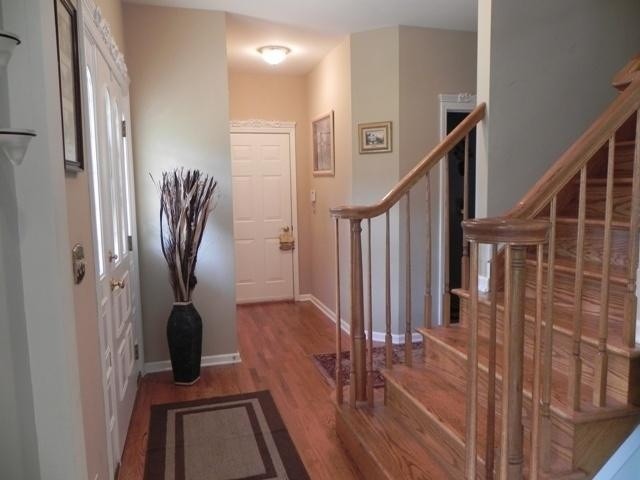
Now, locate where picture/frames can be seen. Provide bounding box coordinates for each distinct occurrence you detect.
[309,110,335,177]
[357,121,393,154]
[54,1,84,172]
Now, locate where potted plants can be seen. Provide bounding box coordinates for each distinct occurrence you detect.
[147,168,221,386]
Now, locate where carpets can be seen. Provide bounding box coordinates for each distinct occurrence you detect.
[313,340,424,391]
[142,388,311,480]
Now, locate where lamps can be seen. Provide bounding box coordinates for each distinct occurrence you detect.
[0,30,36,167]
[257,45,292,66]
